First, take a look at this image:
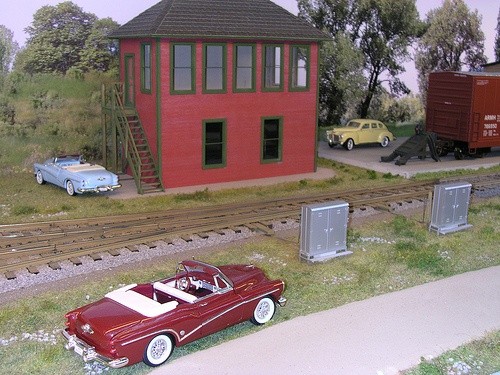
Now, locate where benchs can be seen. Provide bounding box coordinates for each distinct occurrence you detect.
[153,282,197,306]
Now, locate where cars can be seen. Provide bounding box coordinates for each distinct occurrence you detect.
[59,258,287,369]
[34,154,122,196]
[326,119,394,151]
